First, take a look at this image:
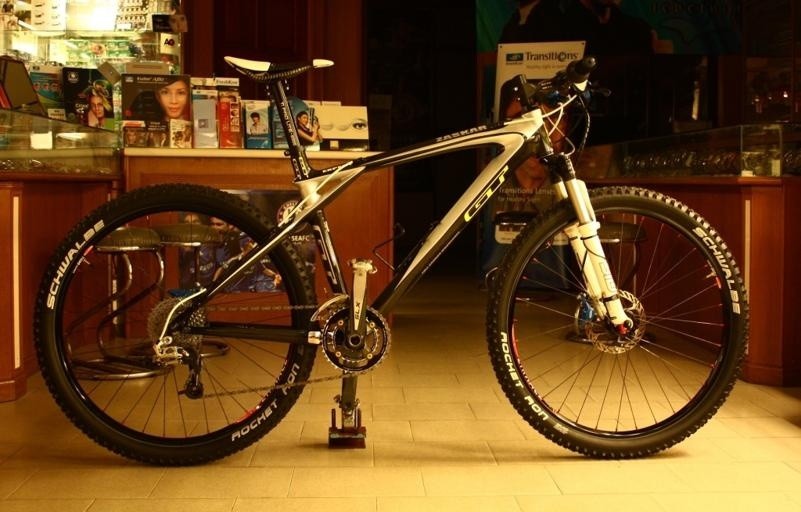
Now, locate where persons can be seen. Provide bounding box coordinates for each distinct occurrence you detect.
[172,125,192,149]
[494,97,567,215]
[82,91,112,128]
[295,109,323,146]
[247,111,268,135]
[76,77,112,102]
[181,212,285,294]
[124,128,137,144]
[159,131,168,147]
[146,131,155,147]
[144,77,187,129]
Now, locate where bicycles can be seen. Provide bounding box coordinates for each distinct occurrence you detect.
[30,55,752,469]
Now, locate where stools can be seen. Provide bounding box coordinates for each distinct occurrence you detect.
[484,211,557,301]
[155,219,229,359]
[569,221,655,346]
[73,227,171,380]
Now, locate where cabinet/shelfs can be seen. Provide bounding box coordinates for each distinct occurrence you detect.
[579,122,801,176]
[0,106,119,175]
